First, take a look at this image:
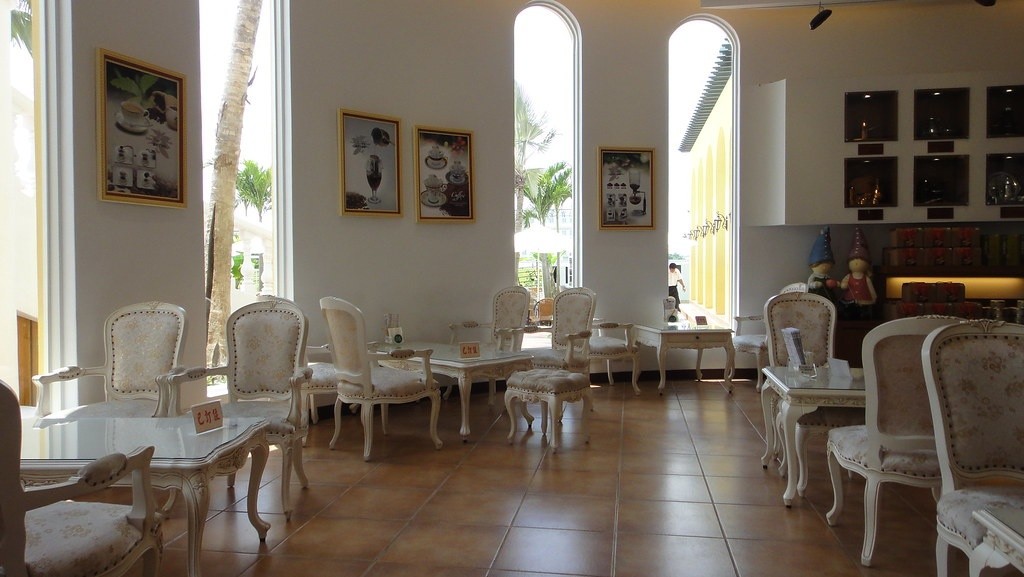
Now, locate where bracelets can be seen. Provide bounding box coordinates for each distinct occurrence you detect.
[683,286,685,287]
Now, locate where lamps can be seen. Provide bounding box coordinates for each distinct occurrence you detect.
[809,2,832,30]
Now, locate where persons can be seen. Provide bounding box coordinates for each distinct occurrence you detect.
[806,226,877,315]
[668,262,686,312]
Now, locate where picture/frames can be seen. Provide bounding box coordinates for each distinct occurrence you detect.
[95,47,189,210]
[336,107,404,219]
[413,123,477,225]
[597,145,657,231]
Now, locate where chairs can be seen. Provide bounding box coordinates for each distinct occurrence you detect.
[441,286,537,405]
[0,379,165,577]
[319,296,443,462]
[726,282,809,392]
[31,301,188,418]
[163,298,312,543]
[922,319,1024,577]
[589,317,643,396]
[505,286,597,453]
[761,292,853,497]
[826,313,965,567]
[301,342,355,423]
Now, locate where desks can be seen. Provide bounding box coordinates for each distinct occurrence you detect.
[371,341,534,437]
[760,367,864,507]
[631,321,737,394]
[19,411,272,577]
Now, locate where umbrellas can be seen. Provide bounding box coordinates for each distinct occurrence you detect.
[514,223,572,300]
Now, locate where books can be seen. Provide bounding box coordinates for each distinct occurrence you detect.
[782,327,805,365]
